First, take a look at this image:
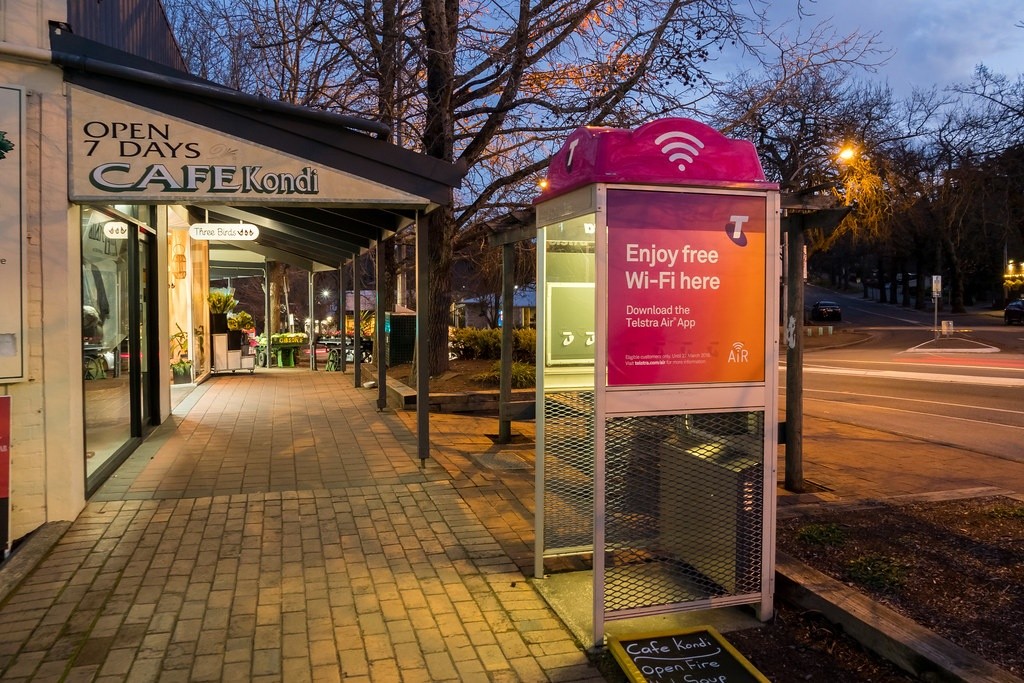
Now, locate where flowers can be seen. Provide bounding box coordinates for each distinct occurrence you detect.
[321,329,370,338]
[242,327,255,333]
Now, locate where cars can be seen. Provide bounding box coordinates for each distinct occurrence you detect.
[1004,300,1024,322]
[811,301,842,322]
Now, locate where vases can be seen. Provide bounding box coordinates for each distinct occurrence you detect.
[242,333,248,345]
[249,333,256,354]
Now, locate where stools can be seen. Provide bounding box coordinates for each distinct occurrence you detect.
[114,350,143,377]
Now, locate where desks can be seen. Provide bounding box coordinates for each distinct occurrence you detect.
[316,341,373,371]
[83,347,110,378]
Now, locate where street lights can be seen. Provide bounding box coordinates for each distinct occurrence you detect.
[783,146,853,344]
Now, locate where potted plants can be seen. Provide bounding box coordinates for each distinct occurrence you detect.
[227,310,252,350]
[170,323,204,384]
[207,290,236,333]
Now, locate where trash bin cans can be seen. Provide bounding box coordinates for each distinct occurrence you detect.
[941,321,953,335]
